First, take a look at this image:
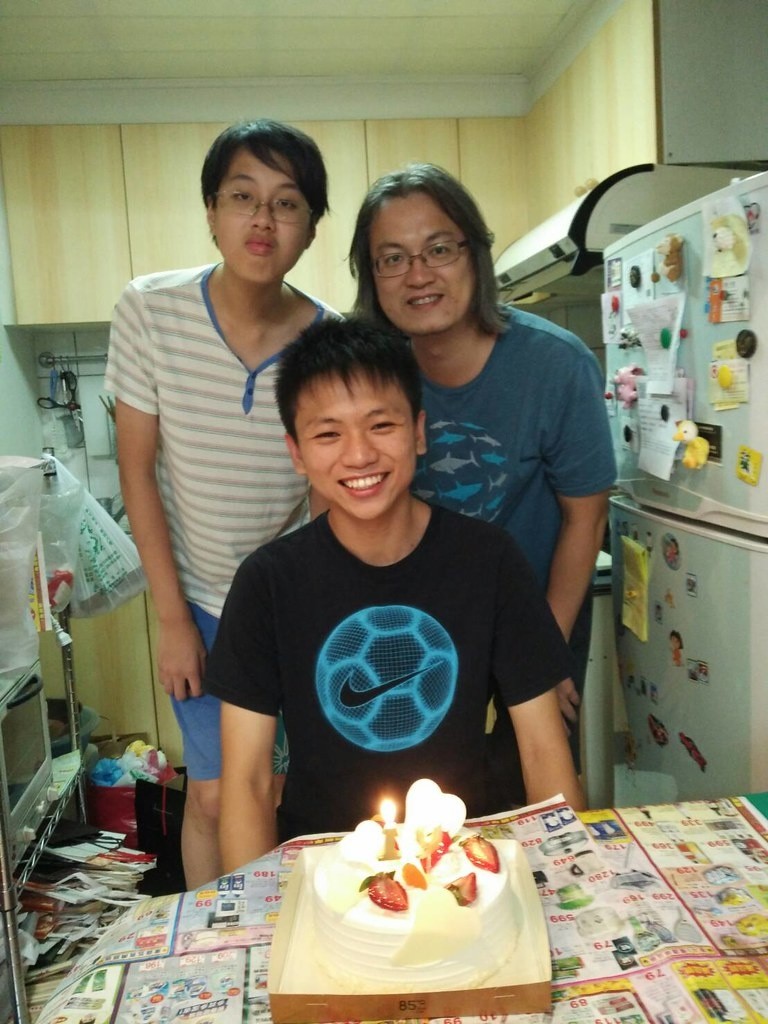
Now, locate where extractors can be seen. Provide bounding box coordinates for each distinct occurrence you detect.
[493,164,762,302]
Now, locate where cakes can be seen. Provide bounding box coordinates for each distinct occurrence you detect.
[304,779,524,992]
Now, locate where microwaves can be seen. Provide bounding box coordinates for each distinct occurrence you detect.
[0,657,57,873]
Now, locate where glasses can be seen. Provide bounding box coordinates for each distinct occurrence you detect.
[369,241,469,278]
[212,190,314,225]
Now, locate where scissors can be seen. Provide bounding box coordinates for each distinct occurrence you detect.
[38,372,95,431]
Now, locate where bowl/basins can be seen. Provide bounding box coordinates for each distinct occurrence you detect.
[49,705,99,758]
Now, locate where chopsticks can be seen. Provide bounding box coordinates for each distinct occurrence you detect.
[99,395,115,420]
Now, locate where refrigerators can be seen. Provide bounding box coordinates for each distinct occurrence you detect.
[602,172,768,808]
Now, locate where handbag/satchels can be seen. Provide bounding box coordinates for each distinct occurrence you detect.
[79,715,188,898]
[0,453,149,677]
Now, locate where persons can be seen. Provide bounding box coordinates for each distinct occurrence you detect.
[198,309,590,880]
[102,115,364,896]
[345,159,622,819]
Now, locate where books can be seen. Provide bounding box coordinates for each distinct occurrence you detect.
[15,815,162,987]
